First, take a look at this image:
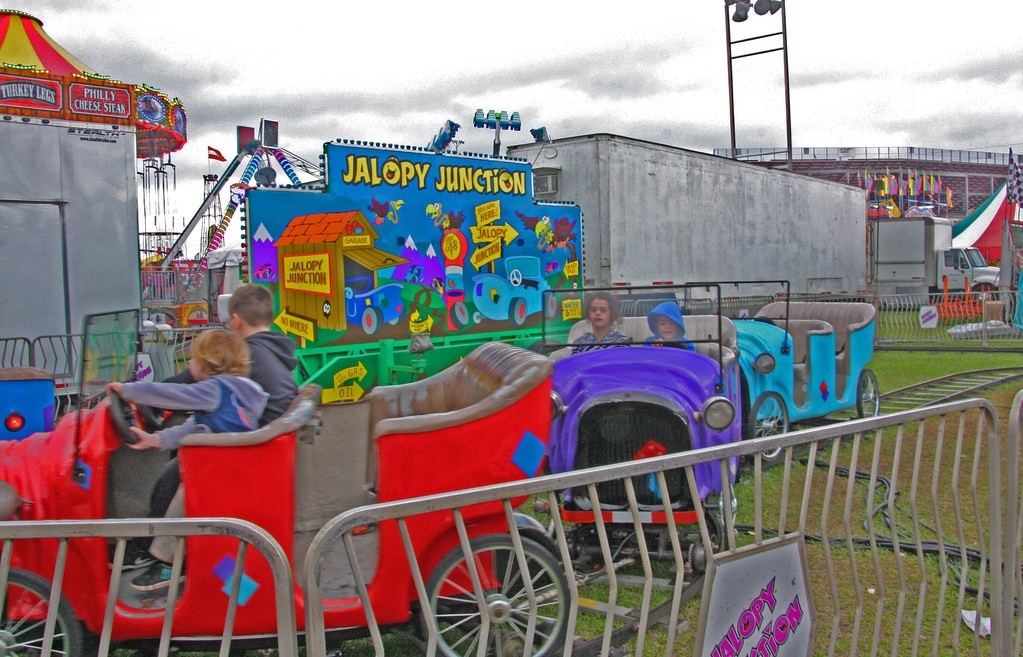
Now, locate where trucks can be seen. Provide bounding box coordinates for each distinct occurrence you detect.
[868,216,1000,315]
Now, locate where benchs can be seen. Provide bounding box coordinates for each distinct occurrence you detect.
[752,300,876,375]
[179,383,323,490]
[549,315,740,373]
[369,340,555,494]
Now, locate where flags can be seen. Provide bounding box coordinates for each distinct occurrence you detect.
[846,168,953,207]
[1006,147,1023,209]
[208,147,226,162]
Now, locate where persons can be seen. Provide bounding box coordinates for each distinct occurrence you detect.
[106,328,270,591]
[639,302,697,353]
[106,284,297,571]
[572,293,631,354]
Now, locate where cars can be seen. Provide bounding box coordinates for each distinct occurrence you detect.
[681,278,881,465]
[540,281,745,574]
[1,337,572,657]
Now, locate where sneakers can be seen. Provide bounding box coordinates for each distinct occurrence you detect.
[109,539,159,573]
[128,561,185,591]
